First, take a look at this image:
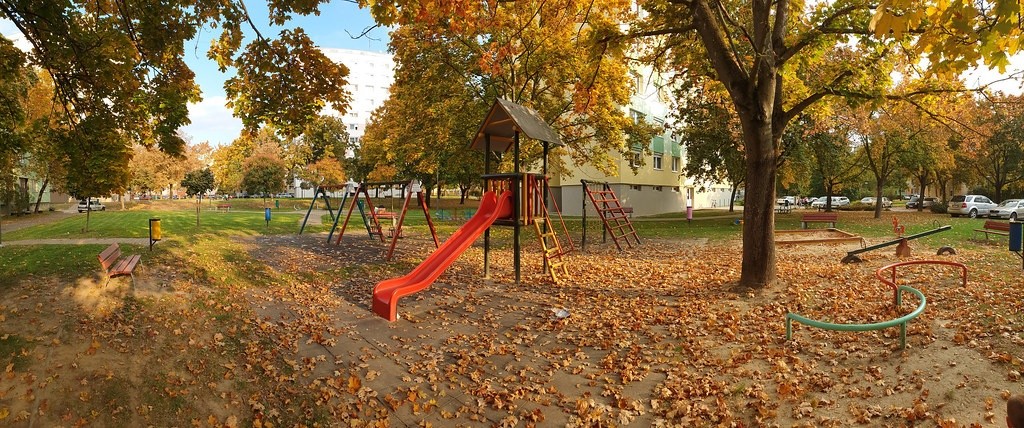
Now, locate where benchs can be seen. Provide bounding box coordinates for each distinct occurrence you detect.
[800,215,838,229]
[97,242,144,289]
[973,220,1011,248]
[367,210,399,226]
[215,202,232,211]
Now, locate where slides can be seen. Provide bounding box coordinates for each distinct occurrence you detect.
[371,188,512,323]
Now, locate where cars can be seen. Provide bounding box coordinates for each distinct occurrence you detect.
[903,194,938,210]
[78,199,106,213]
[222,192,292,199]
[777,195,850,210]
[988,198,1024,221]
[946,194,998,219]
[860,196,893,208]
